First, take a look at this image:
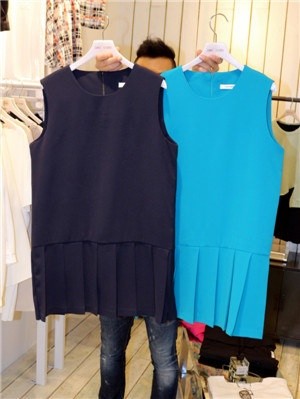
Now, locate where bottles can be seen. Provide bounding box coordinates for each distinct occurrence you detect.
[228,351,250,382]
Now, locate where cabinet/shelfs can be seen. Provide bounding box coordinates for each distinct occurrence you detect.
[263,252,300,346]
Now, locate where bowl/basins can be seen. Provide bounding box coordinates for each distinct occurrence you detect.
[221,365,261,382]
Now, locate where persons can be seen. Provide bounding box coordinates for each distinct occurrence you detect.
[94,38,224,399]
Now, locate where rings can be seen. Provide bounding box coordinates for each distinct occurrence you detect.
[209,67,213,71]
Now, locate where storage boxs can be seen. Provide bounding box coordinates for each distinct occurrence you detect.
[270,237,289,262]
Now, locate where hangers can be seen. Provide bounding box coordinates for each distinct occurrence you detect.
[276,97,300,123]
[40,11,167,89]
[182,13,277,91]
[0,80,41,127]
